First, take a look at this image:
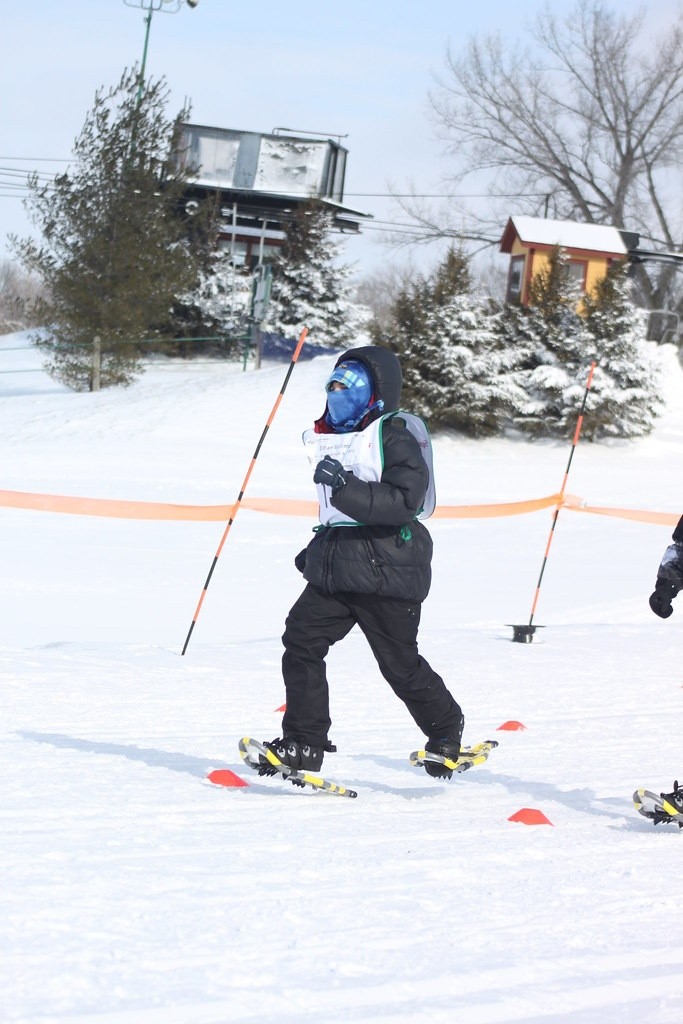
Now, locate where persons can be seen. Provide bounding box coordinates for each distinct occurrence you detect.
[649,512,683,814]
[262,344,465,773]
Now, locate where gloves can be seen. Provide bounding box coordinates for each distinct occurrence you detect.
[295,548,307,573]
[313,455,348,492]
[649,579,680,618]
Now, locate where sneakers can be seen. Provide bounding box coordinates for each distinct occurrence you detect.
[660,780,683,814]
[259,736,336,772]
[424,714,464,776]
